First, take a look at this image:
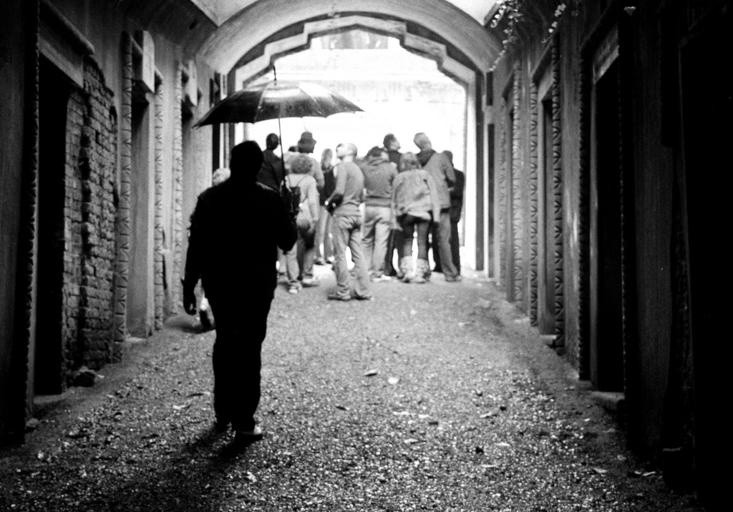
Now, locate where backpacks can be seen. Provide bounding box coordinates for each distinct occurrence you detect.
[287,173,307,212]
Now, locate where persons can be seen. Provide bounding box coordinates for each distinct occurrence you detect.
[255,130,464,301]
[182,139,301,443]
[197,166,232,330]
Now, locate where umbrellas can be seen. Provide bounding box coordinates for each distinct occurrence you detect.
[187,61,366,185]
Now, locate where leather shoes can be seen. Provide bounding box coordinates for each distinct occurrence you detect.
[235,426,262,441]
